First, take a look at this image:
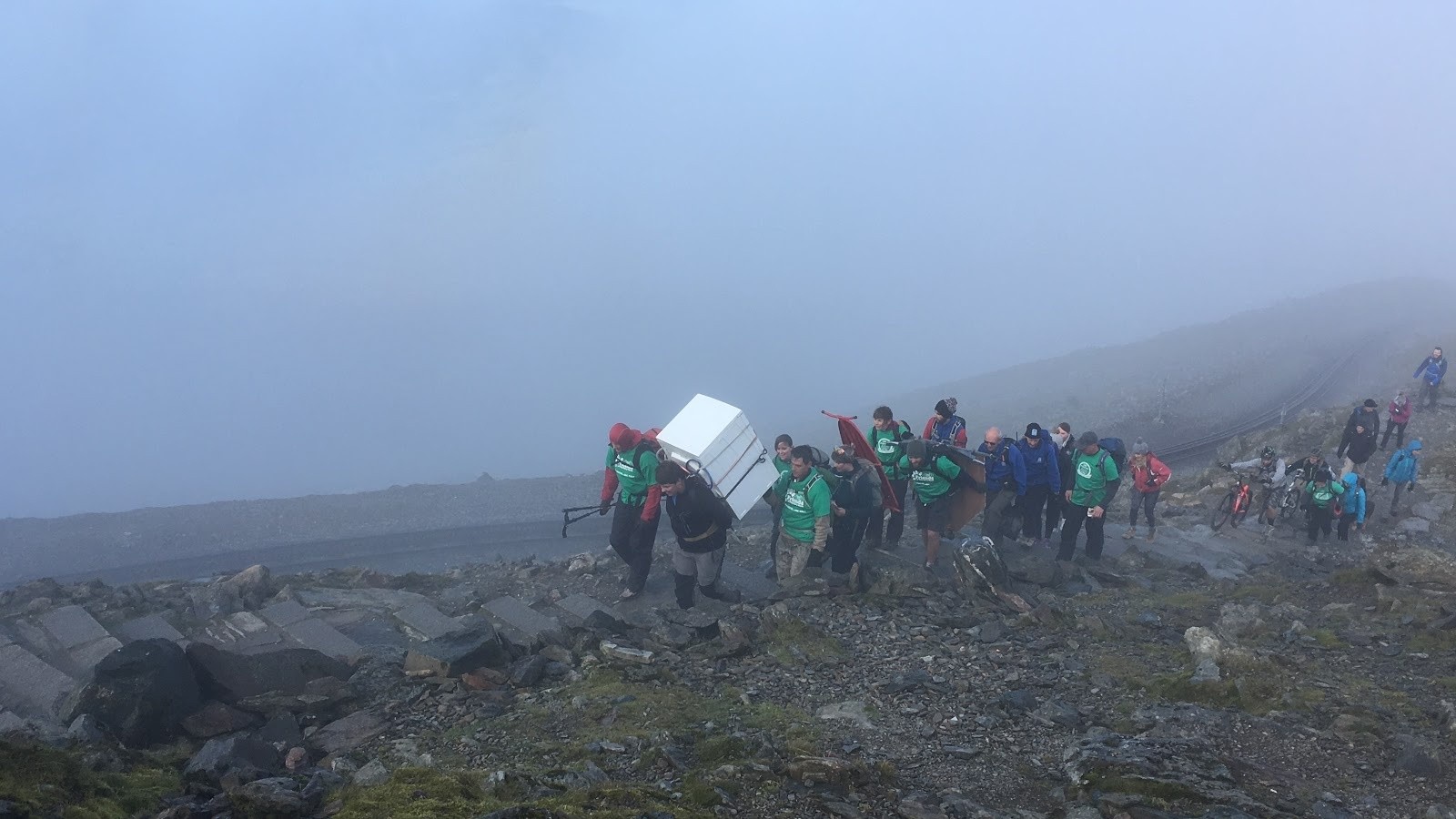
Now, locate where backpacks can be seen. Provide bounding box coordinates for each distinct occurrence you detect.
[908,439,965,496]
[872,420,912,484]
[1147,451,1159,480]
[786,466,843,527]
[1075,438,1127,476]
[774,447,830,468]
[1356,476,1366,502]
[931,416,966,445]
[611,427,666,514]
[851,457,883,507]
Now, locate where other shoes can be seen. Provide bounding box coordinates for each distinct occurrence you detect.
[1060,518,1067,531]
[619,588,641,602]
[1378,444,1385,450]
[1017,534,1035,548]
[734,589,741,605]
[1052,528,1059,533]
[1262,524,1274,537]
[1147,528,1155,543]
[1121,527,1137,539]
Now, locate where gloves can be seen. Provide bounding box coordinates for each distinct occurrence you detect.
[1144,479,1155,487]
[1408,483,1414,491]
[630,521,651,554]
[600,501,611,515]
[1336,505,1340,512]
[1133,473,1135,480]
[1382,477,1388,486]
[1357,523,1361,530]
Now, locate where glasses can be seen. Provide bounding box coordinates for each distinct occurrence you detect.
[935,411,942,415]
[833,445,853,462]
[984,440,999,445]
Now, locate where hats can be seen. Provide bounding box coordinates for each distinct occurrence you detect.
[1364,399,1378,408]
[935,398,958,418]
[1315,471,1327,482]
[1133,437,1149,455]
[1310,449,1321,456]
[1259,446,1276,457]
[1077,431,1098,450]
[1025,423,1042,438]
[906,440,926,458]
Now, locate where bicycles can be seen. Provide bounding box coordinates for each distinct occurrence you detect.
[1258,467,1306,525]
[1210,464,1272,532]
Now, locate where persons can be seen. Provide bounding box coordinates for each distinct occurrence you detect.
[1122,437,1173,544]
[1218,392,1421,541]
[656,461,743,609]
[599,423,664,600]
[1412,346,1448,412]
[761,398,966,589]
[979,422,1120,563]
